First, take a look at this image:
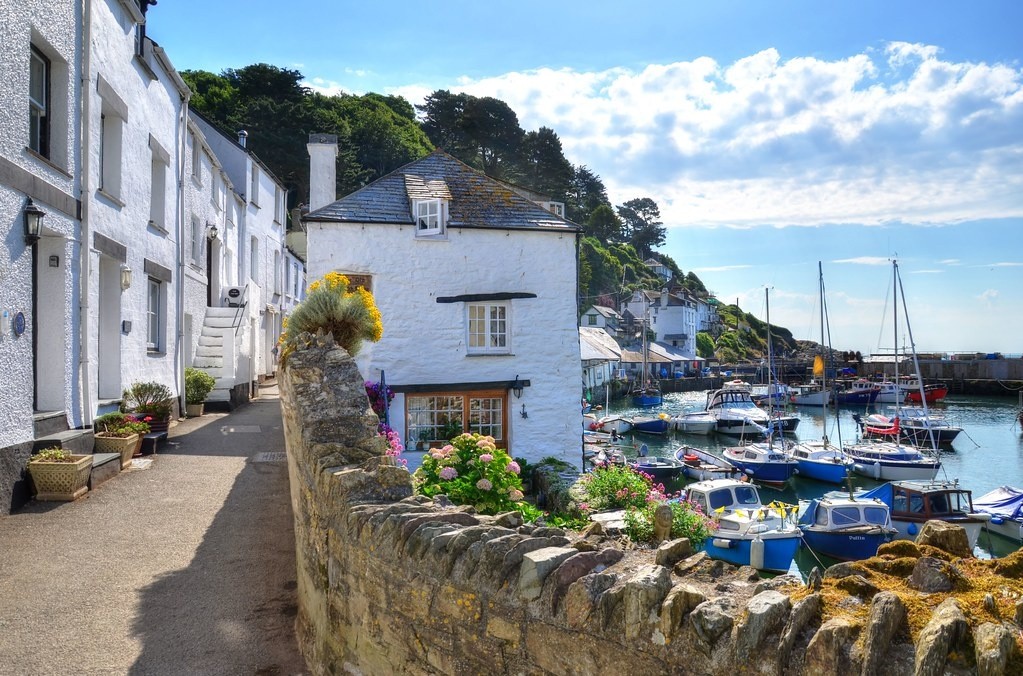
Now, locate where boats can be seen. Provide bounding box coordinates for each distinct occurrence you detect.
[769,466,899,564]
[747,359,797,407]
[629,417,670,436]
[892,335,948,403]
[822,370,881,406]
[584,413,597,431]
[597,384,633,436]
[679,478,800,575]
[669,411,716,438]
[874,382,907,403]
[824,478,989,554]
[962,486,1023,542]
[625,444,685,482]
[675,444,743,485]
[786,380,830,406]
[707,388,769,435]
[722,379,762,404]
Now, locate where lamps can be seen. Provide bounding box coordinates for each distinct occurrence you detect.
[509,382,523,399]
[120,264,132,289]
[206,220,217,239]
[23,195,45,246]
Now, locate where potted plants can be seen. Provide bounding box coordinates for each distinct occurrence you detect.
[28,445,93,500]
[418,430,430,450]
[184,367,215,416]
[93,427,139,470]
[120,381,175,433]
[514,457,536,493]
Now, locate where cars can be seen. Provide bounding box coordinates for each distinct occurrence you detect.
[687,367,711,376]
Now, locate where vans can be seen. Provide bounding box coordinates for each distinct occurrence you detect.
[986,354,1002,359]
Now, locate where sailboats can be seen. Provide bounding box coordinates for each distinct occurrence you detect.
[841,260,942,482]
[628,314,663,406]
[723,285,799,490]
[782,257,854,484]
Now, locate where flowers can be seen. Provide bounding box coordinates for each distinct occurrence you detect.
[123,416,150,433]
[361,381,719,547]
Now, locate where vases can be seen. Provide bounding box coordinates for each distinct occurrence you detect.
[134,434,143,456]
